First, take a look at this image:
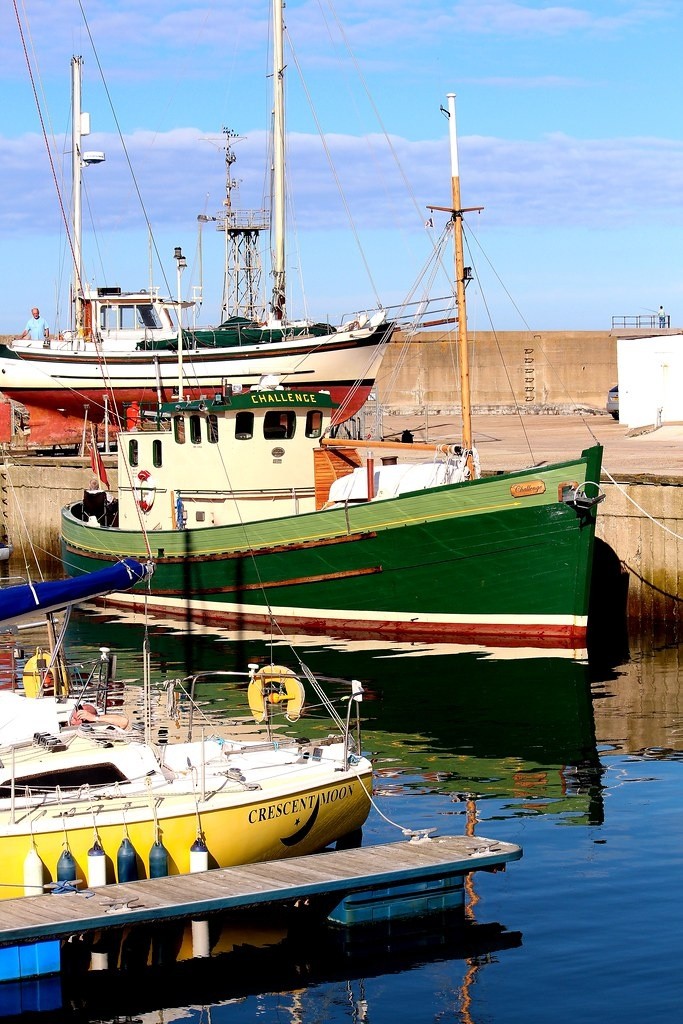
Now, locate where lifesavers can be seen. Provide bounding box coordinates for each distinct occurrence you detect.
[246,664,306,724]
[22,652,69,700]
[133,469,157,513]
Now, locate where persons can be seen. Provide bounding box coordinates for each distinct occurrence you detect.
[658,306,666,329]
[70,704,129,730]
[16,308,49,340]
[89,480,100,491]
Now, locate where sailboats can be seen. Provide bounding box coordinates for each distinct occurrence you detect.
[0,0,394,431]
[0,0,374,901]
[59,91,606,669]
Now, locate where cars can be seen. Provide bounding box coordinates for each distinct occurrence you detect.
[607,386,619,420]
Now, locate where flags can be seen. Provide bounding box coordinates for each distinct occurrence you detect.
[90,429,110,490]
